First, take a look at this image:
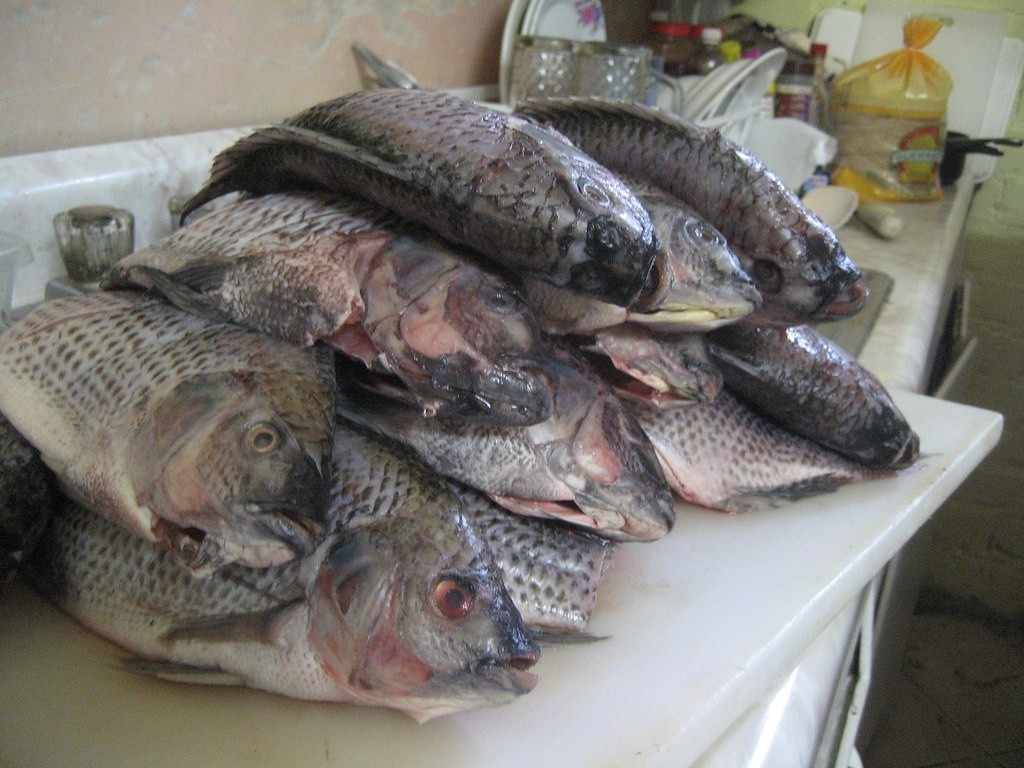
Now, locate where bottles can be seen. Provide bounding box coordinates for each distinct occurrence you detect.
[686,28,726,76]
[802,44,830,195]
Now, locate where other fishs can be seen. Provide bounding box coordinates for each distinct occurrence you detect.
[0,89,921,722]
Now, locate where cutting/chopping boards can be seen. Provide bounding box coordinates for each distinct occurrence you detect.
[0,388,1002,768]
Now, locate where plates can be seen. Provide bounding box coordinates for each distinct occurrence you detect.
[498,0,529,104]
[802,187,858,231]
[520,0,606,43]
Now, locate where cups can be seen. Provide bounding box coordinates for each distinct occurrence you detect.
[507,36,578,108]
[572,43,684,117]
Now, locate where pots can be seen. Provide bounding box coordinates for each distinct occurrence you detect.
[940,131,1023,186]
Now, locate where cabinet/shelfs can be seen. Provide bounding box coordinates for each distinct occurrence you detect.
[0,163,975,768]
